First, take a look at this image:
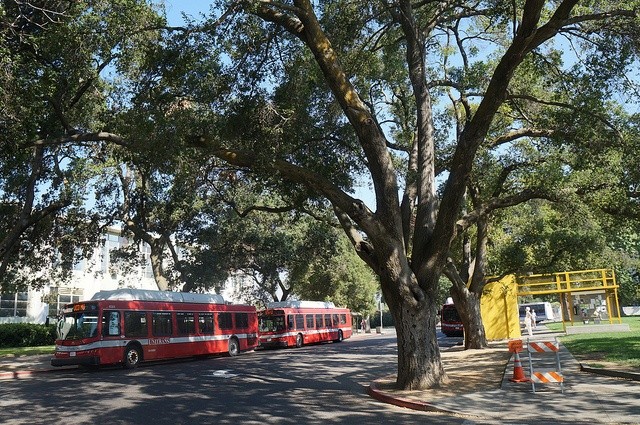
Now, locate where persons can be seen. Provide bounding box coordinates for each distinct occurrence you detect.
[525,307,533,339]
[530,309,538,328]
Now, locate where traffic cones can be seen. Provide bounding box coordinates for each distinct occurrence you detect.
[509,347,531,383]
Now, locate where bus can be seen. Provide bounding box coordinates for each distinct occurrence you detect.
[50,288,260,371]
[256,299,352,348]
[520,301,555,325]
[439,298,465,337]
